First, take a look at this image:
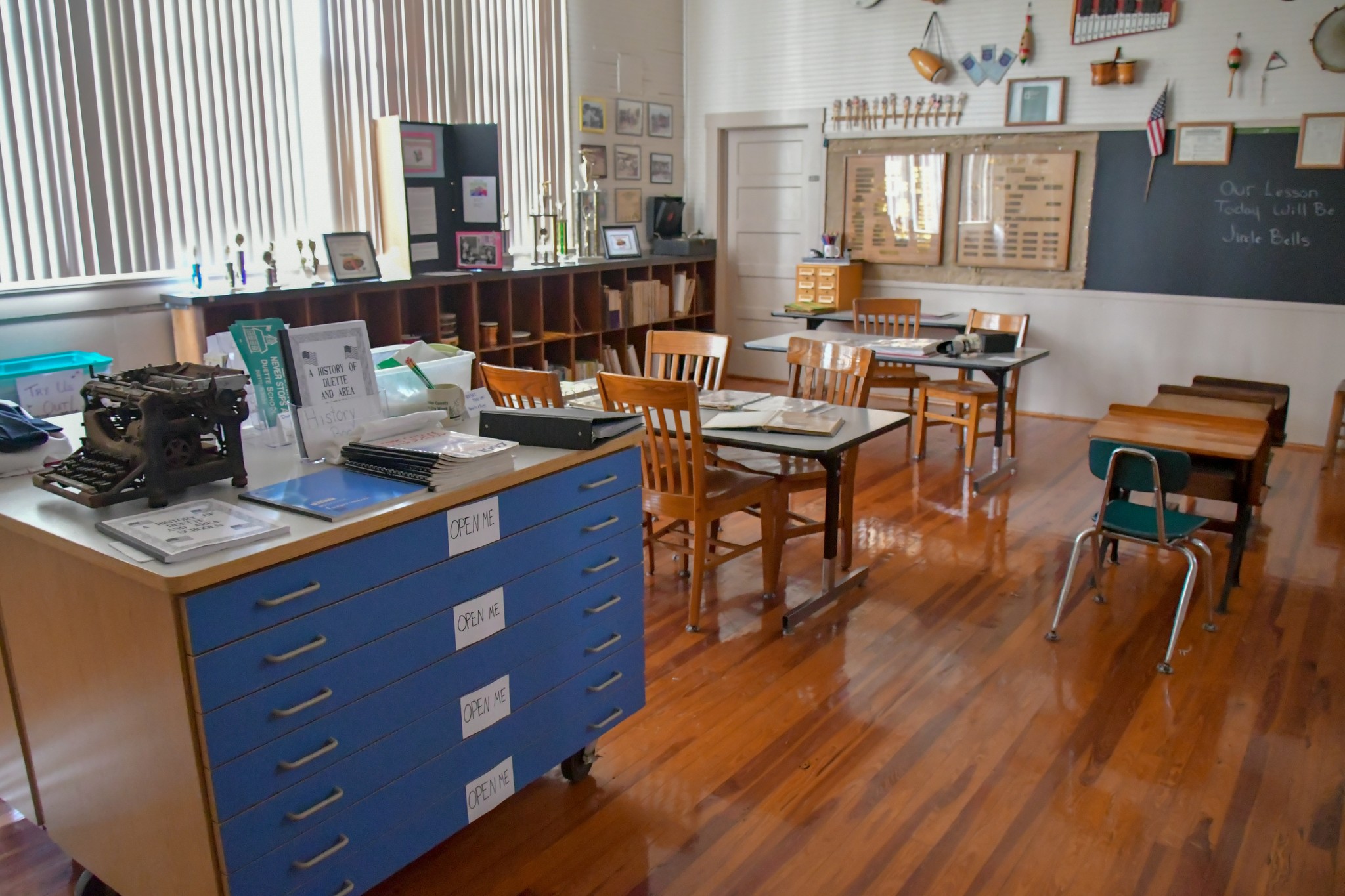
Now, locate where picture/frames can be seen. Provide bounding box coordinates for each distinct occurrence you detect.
[1005,76,1066,126]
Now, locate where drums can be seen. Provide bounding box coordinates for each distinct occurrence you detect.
[1090,60,1114,85]
[1116,59,1136,85]
[907,47,947,84]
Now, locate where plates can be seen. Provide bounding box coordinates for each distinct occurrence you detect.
[512,331,531,338]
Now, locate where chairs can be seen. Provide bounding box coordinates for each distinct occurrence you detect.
[644,329,732,392]
[852,297,930,437]
[1043,439,1217,674]
[705,336,877,571]
[596,370,780,633]
[479,362,565,410]
[910,309,1030,473]
[1320,378,1345,472]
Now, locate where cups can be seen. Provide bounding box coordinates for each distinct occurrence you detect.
[1115,57,1138,86]
[426,383,466,427]
[1091,60,1111,86]
[824,245,840,258]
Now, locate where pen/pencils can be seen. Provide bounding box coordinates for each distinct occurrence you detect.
[821,231,839,245]
[405,357,435,389]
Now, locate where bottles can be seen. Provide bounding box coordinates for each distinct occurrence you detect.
[845,249,852,259]
[909,48,948,83]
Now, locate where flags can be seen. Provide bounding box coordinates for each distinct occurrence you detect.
[1146,86,1166,158]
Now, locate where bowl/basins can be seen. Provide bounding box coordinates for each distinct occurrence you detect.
[403,335,420,343]
[439,313,459,348]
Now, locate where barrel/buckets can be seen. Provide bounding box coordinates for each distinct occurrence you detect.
[481,322,499,344]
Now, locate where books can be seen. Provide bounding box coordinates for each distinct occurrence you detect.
[863,336,947,359]
[516,270,712,380]
[236,466,428,524]
[95,497,291,565]
[341,424,522,492]
[511,379,657,419]
[784,301,834,313]
[785,309,836,316]
[695,387,838,418]
[700,408,845,437]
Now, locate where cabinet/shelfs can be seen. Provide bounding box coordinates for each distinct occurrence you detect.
[160,252,716,383]
[0,408,647,895]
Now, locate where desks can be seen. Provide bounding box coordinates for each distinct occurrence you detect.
[743,329,1051,499]
[770,309,977,417]
[640,390,912,635]
[1089,375,1289,614]
